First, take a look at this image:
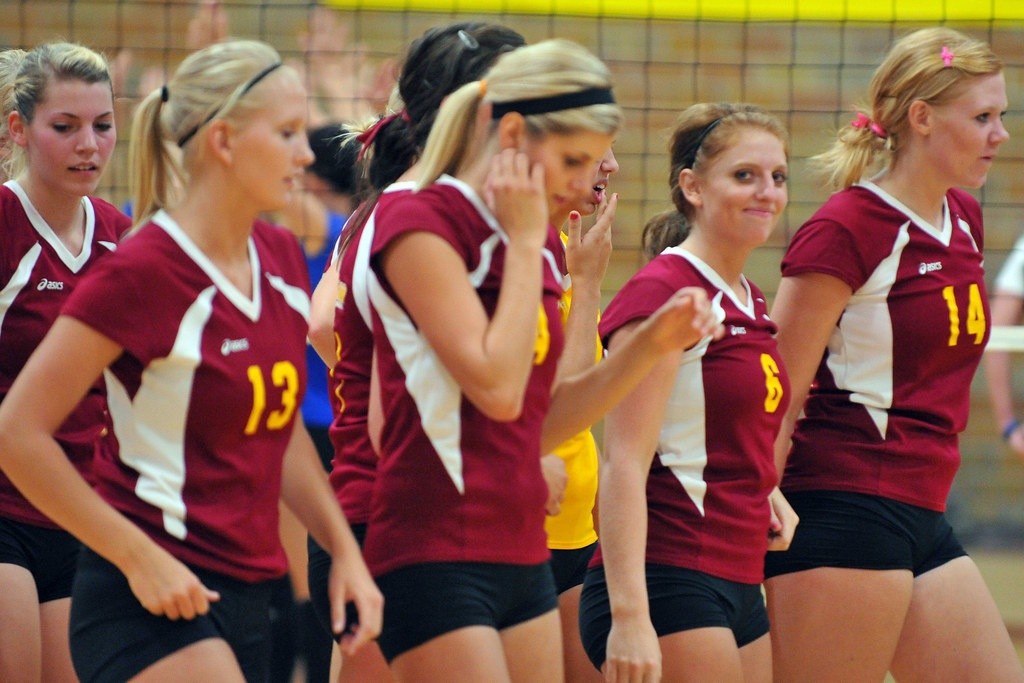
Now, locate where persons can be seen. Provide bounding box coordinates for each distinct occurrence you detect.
[275,123,362,683]
[308,22,727,683]
[0,39,385,683]
[983,237,1024,456]
[0,43,131,683]
[578,101,800,683]
[770,26,1024,683]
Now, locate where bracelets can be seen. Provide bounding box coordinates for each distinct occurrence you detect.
[1003,420,1020,441]
